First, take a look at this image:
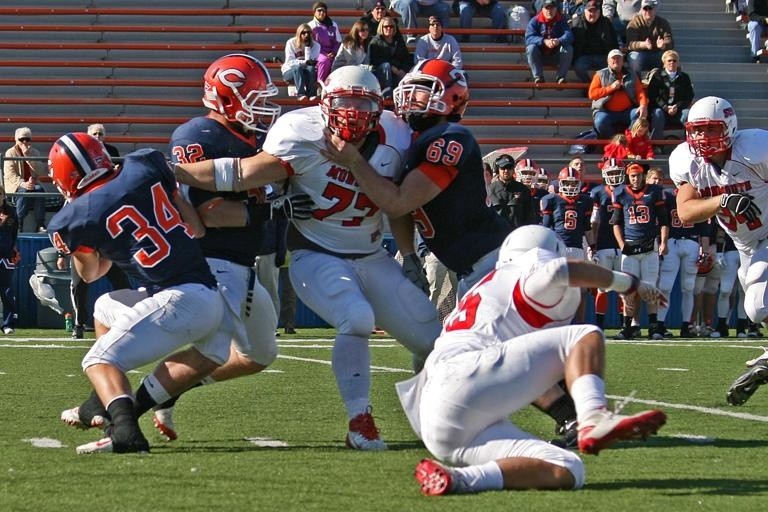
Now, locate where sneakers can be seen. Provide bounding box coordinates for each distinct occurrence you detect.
[612,325,640,339]
[735,326,746,338]
[550,418,578,450]
[2,326,15,335]
[647,323,672,341]
[578,404,666,456]
[141,375,179,440]
[726,358,768,407]
[413,456,458,497]
[63,313,95,339]
[534,77,545,91]
[344,413,390,452]
[555,77,568,90]
[274,327,297,338]
[75,433,151,456]
[60,405,111,428]
[746,329,761,338]
[295,93,319,103]
[679,323,729,338]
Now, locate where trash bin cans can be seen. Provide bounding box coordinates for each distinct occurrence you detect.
[16,233,71,329]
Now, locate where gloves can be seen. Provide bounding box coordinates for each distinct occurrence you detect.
[718,192,762,224]
[622,269,669,310]
[269,190,316,222]
[591,251,601,266]
[399,252,433,297]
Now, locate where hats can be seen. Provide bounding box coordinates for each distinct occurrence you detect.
[492,155,514,172]
[428,15,443,25]
[543,0,558,9]
[606,47,624,60]
[626,161,644,174]
[369,1,386,10]
[640,0,656,9]
[585,1,599,11]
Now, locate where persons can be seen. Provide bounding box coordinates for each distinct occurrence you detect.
[2,127,50,234]
[45,129,228,456]
[90,53,316,442]
[392,224,670,497]
[163,66,444,451]
[0,184,17,337]
[280,0,768,340]
[57,247,133,340]
[725,348,767,408]
[316,57,585,451]
[83,123,121,171]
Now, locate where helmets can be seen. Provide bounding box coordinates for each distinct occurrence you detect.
[202,53,283,133]
[495,224,569,269]
[515,158,539,188]
[696,250,715,277]
[537,168,550,189]
[392,58,472,124]
[47,131,124,203]
[685,95,739,158]
[600,157,626,188]
[557,167,582,199]
[318,65,385,147]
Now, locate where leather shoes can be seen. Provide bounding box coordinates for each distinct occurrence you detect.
[16,217,24,233]
[36,224,48,233]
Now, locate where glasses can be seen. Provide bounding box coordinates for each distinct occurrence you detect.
[18,137,33,143]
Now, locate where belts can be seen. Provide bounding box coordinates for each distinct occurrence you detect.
[670,234,701,240]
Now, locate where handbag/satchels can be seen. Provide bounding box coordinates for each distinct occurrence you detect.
[505,5,530,29]
[568,127,600,154]
